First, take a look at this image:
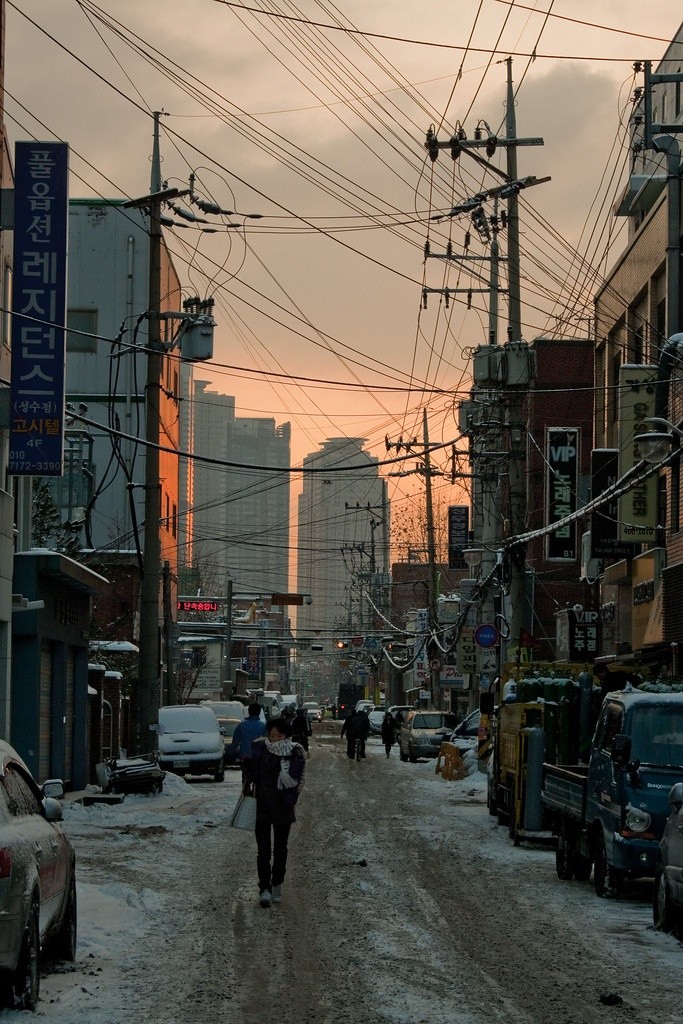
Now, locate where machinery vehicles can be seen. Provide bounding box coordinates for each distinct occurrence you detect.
[476,680,580,846]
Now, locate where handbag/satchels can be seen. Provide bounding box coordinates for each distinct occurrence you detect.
[229,778,257,831]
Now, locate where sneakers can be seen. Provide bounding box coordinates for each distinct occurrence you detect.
[259,888,271,905]
[272,885,282,903]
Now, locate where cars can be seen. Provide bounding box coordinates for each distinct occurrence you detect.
[157,689,302,782]
[648,778,683,935]
[301,701,336,722]
[448,704,498,743]
[1,737,80,1011]
[398,707,461,763]
[355,697,417,738]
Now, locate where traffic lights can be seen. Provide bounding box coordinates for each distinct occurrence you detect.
[337,641,349,649]
[387,643,392,650]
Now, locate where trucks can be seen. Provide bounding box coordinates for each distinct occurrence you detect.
[541,682,683,900]
[336,681,366,720]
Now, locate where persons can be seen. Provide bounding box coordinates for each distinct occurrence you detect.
[230,703,405,801]
[241,717,306,909]
